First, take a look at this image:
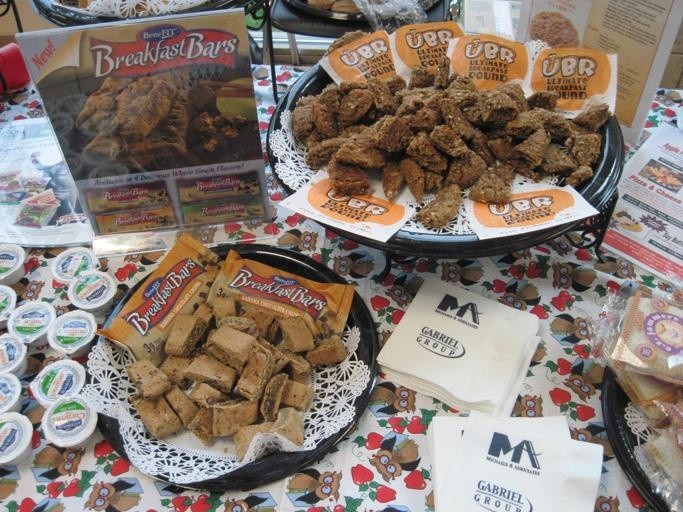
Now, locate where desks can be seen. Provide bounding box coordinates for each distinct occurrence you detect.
[263,0,455,68]
[31,0,273,65]
[1,64,678,511]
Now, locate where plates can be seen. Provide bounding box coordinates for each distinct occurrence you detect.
[266,34,623,280]
[32,0,240,27]
[282,0,442,23]
[601,366,670,511]
[93,243,378,492]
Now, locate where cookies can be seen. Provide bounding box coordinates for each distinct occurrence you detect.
[306,0,361,16]
[126,298,346,461]
[293,58,611,227]
[72,72,238,167]
[601,294,683,491]
